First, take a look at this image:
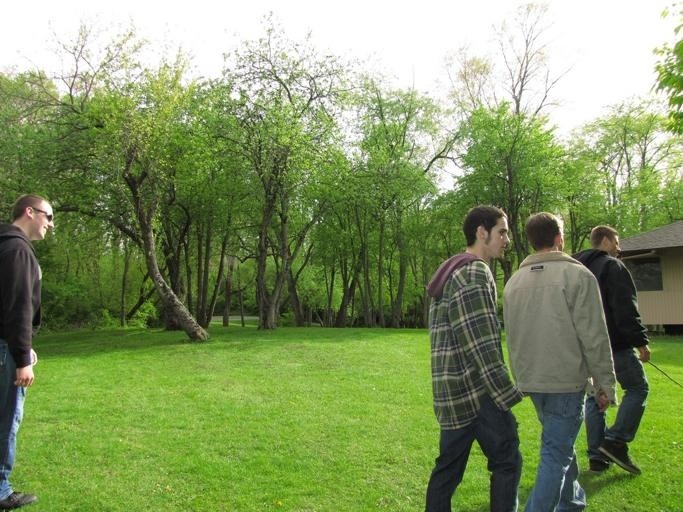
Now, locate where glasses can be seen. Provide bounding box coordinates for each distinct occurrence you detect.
[32,207,52,222]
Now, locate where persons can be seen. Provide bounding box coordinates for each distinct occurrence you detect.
[0,194,54,509]
[502,212,617,512]
[568,225,650,475]
[426,206,523,512]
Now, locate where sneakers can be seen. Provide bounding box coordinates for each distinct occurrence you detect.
[598,440,641,475]
[0,491,36,509]
[588,459,608,474]
